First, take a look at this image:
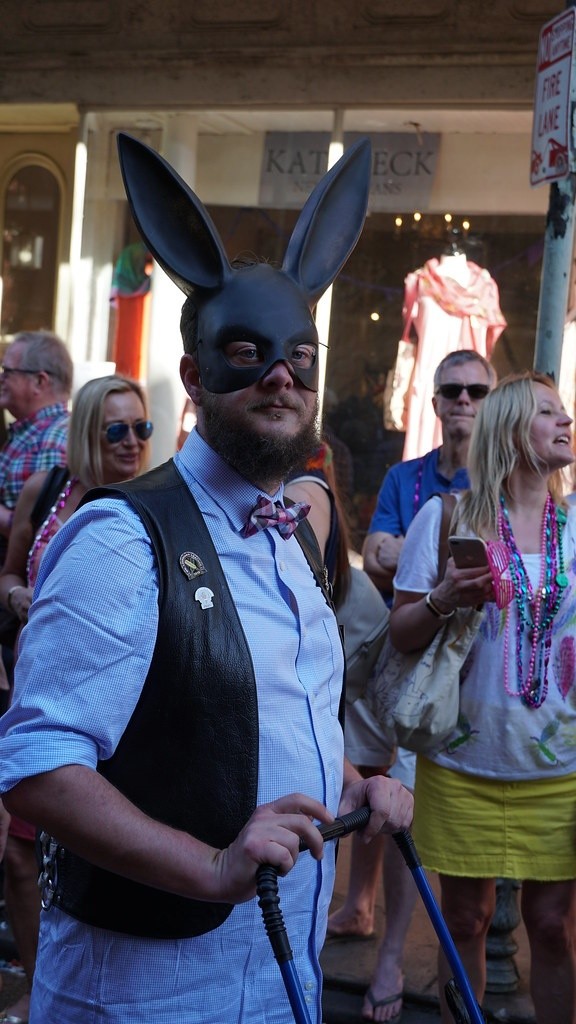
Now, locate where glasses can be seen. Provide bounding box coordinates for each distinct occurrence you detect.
[436,383,492,400]
[1,366,51,376]
[101,422,153,442]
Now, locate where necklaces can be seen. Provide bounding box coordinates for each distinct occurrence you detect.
[497,494,569,709]
[27,482,75,588]
[413,456,424,515]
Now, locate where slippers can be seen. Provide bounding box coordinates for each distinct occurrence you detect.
[320,935,375,948]
[358,988,403,1024]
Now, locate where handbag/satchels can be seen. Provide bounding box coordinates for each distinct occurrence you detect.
[344,492,484,755]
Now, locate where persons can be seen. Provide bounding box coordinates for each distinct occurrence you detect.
[0,373,155,1024]
[0,329,76,564]
[324,466,416,1024]
[0,261,419,1024]
[326,350,499,943]
[388,371,576,1024]
[284,444,353,615]
[384,254,507,462]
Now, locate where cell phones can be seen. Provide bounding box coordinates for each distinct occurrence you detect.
[446,536,490,574]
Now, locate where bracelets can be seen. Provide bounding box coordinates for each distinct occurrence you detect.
[7,586,21,608]
[426,590,455,620]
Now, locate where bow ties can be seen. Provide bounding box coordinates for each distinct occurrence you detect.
[240,494,313,543]
